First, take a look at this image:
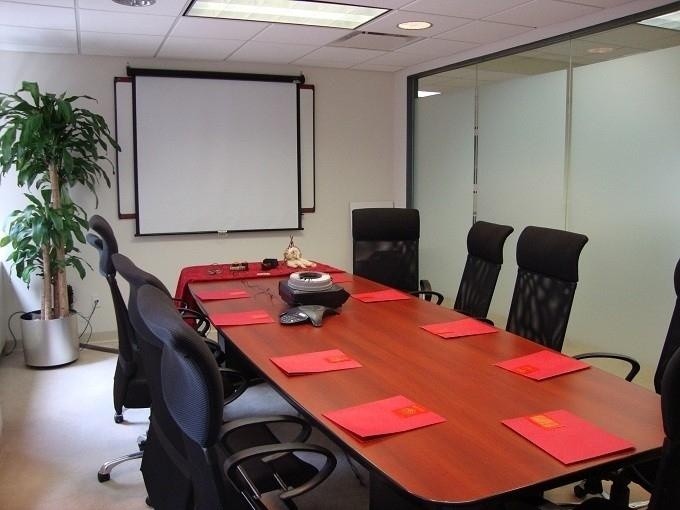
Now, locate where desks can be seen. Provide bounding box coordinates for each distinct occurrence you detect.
[177,261,667,509]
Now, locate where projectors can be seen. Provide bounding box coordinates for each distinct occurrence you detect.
[277,280,351,308]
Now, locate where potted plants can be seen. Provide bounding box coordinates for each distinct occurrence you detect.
[0,79,121,368]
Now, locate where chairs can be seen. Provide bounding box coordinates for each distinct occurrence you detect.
[84,214,222,423]
[99,255,245,485]
[136,287,335,510]
[402,222,513,316]
[472,225,590,354]
[547,262,679,507]
[351,208,433,300]
